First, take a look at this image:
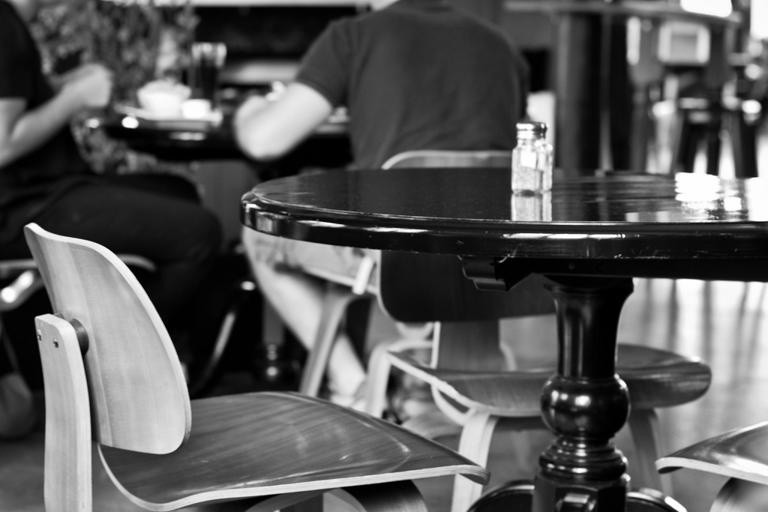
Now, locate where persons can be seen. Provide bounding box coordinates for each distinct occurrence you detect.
[2,1,264,398]
[231,2,532,425]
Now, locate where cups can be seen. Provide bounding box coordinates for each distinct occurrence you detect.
[190,39,227,110]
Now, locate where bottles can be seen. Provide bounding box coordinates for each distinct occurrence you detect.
[510,192,552,223]
[509,122,553,194]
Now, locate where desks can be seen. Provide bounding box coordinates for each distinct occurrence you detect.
[100,118,357,171]
